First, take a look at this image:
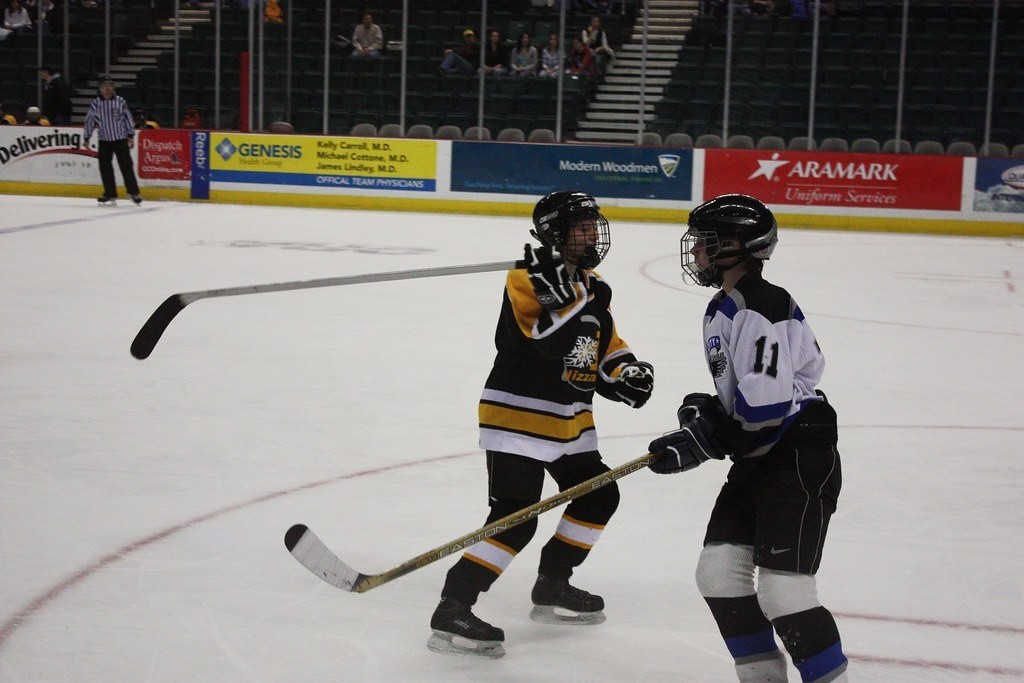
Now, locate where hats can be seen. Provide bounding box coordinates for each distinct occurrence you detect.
[98,76,113,83]
[463,30,474,35]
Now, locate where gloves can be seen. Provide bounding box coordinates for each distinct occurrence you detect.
[677,393,723,429]
[648,416,725,474]
[614,360,654,408]
[525,243,578,312]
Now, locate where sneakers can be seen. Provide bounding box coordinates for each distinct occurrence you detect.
[131,193,142,206]
[97,196,117,206]
[427,598,506,659]
[530,577,606,624]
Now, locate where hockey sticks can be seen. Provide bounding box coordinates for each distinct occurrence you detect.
[129,258,530,361]
[283,452,656,592]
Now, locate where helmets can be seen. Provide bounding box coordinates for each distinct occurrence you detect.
[27,107,41,113]
[680,194,778,289]
[531,191,611,270]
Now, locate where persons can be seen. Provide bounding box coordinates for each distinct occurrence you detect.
[38,66,73,125]
[4,0,54,47]
[341,11,384,88]
[437,28,480,74]
[83,75,142,203]
[476,26,509,81]
[647,193,848,683]
[581,15,616,64]
[118,86,161,129]
[699,0,836,19]
[430,189,653,642]
[0,104,52,126]
[510,31,538,79]
[564,35,596,78]
[539,32,567,79]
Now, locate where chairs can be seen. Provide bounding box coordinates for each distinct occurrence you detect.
[0,0,1024,160]
